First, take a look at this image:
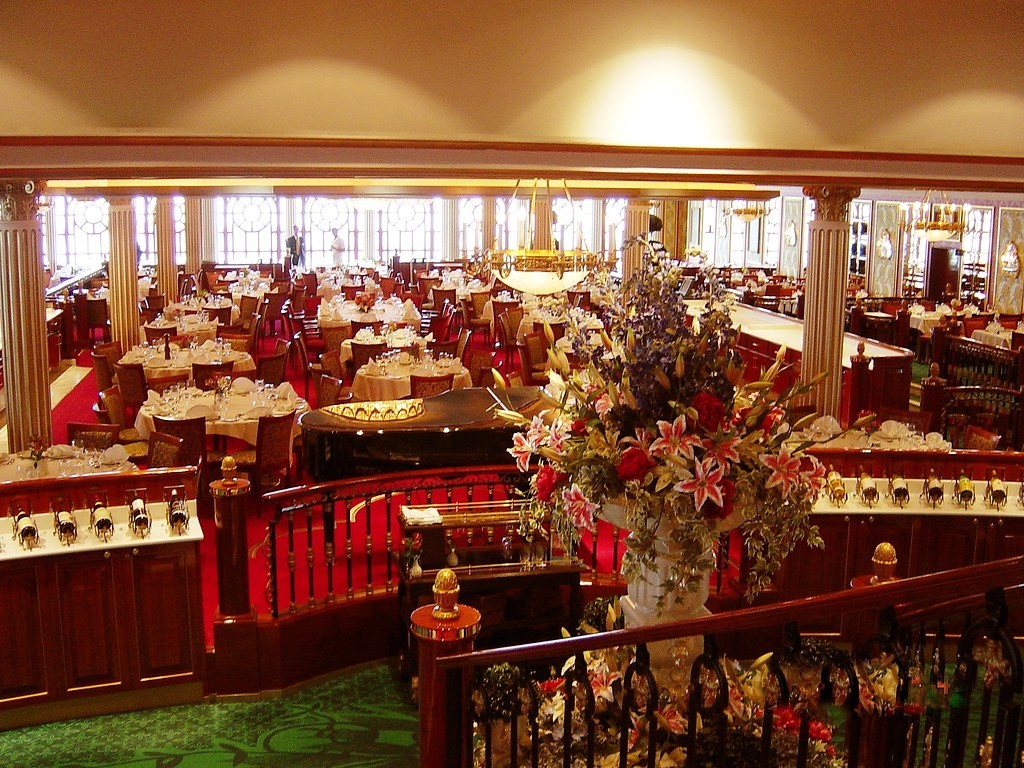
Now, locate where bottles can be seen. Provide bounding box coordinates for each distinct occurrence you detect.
[54,498,76,537]
[959,468,973,501]
[928,468,942,498]
[890,467,908,500]
[827,464,845,498]
[169,489,187,526]
[93,494,111,533]
[990,469,1005,503]
[859,464,877,501]
[15,502,37,541]
[130,490,148,530]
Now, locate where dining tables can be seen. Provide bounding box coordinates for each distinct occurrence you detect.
[0,451,140,484]
[163,302,241,326]
[971,329,1012,348]
[340,336,428,374]
[231,291,266,307]
[896,311,964,334]
[139,321,225,346]
[480,299,517,320]
[316,278,382,300]
[112,348,256,384]
[134,391,313,447]
[352,362,473,401]
[317,300,421,337]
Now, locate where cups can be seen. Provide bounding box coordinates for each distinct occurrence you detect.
[422,348,454,376]
[804,422,833,447]
[134,267,590,365]
[898,423,952,450]
[17,448,107,479]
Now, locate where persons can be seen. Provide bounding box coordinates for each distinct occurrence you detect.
[329,228,345,266]
[286,225,306,266]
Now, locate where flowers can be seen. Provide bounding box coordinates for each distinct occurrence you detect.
[539,597,894,768]
[476,236,879,617]
[204,373,232,390]
[24,435,53,460]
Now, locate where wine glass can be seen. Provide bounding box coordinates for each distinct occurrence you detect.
[376,349,402,376]
[72,437,83,461]
[250,379,278,411]
[162,379,198,414]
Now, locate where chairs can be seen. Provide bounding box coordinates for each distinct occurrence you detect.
[694,274,1024,362]
[66,263,604,518]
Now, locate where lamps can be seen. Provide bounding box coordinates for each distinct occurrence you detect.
[726,200,771,222]
[897,189,970,242]
[462,177,619,295]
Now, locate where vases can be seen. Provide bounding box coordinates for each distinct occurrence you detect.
[33,457,48,478]
[602,500,762,694]
[213,393,227,414]
